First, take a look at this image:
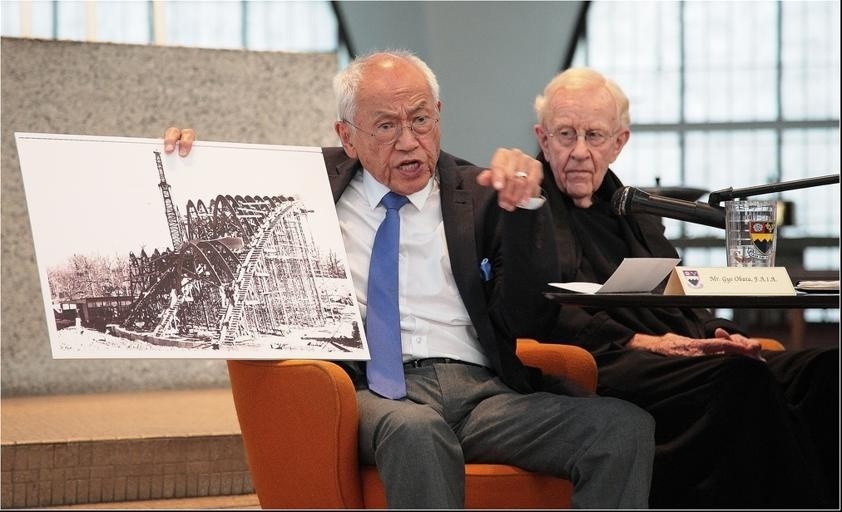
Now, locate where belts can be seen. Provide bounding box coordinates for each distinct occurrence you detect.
[405,358,472,369]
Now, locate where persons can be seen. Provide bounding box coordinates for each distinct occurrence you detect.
[533,68,840,508]
[165,47,657,508]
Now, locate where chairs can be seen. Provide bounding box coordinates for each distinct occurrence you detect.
[227,337,597,509]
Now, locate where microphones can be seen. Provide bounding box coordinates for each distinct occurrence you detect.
[611,185,744,229]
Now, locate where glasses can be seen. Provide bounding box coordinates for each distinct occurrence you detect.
[542,128,614,147]
[345,107,440,146]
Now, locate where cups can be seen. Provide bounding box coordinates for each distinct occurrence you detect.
[724,199,779,268]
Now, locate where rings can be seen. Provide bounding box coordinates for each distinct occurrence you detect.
[515,172,528,179]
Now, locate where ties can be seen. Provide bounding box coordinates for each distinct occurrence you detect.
[365,191,412,401]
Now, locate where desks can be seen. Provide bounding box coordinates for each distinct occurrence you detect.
[543,289,839,308]
